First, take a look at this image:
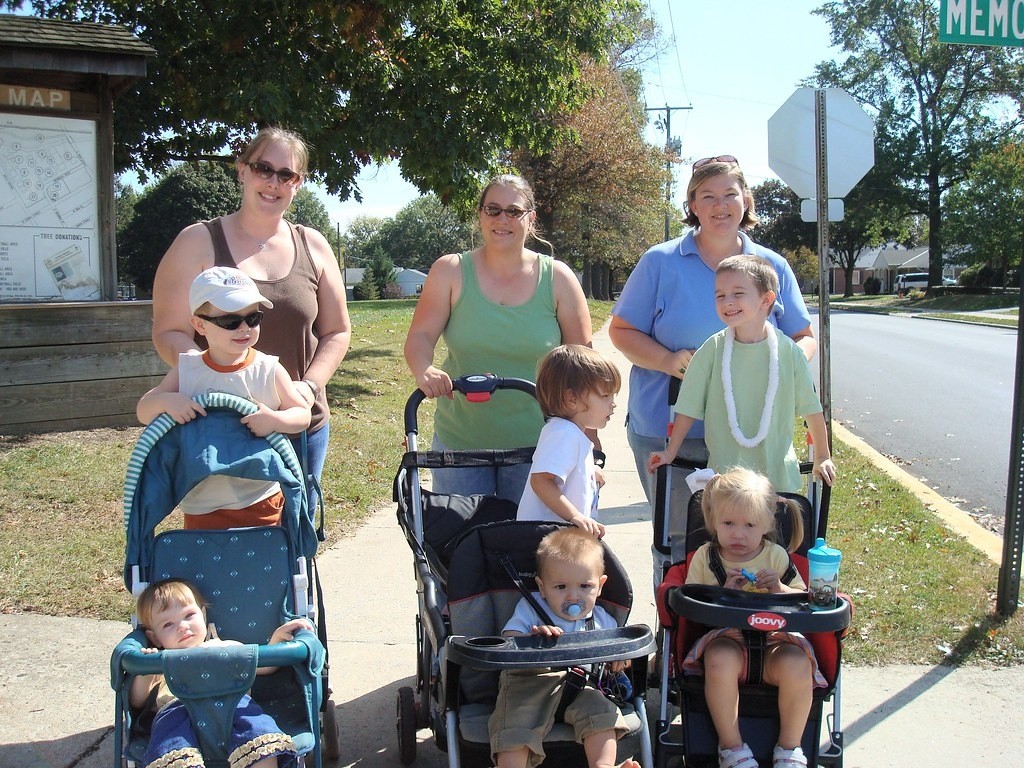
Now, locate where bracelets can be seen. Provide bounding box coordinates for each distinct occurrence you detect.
[302,379,320,400]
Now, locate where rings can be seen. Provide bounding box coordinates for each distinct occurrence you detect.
[680,368,686,373]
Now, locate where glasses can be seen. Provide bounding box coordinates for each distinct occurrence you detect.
[693,155,739,177]
[197,311,264,330]
[481,205,532,218]
[245,161,302,186]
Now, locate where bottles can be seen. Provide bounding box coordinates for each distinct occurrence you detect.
[808,538,842,611]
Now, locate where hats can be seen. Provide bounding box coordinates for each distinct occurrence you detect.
[189,266,274,317]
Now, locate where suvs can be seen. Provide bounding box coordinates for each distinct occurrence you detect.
[894,273,959,296]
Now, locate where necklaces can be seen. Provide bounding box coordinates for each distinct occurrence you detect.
[235,210,279,248]
[721,320,779,448]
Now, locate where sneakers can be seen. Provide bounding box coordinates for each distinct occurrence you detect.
[773,742,808,768]
[718,742,759,768]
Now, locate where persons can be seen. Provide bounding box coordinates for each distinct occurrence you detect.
[487,526,643,768]
[136,265,312,529]
[516,343,623,539]
[129,578,315,768]
[647,254,837,493]
[402,174,608,693]
[683,465,829,768]
[154,128,350,524]
[608,154,818,685]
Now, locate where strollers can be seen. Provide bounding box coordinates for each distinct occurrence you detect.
[110,394,341,768]
[642,351,854,768]
[391,371,662,768]
